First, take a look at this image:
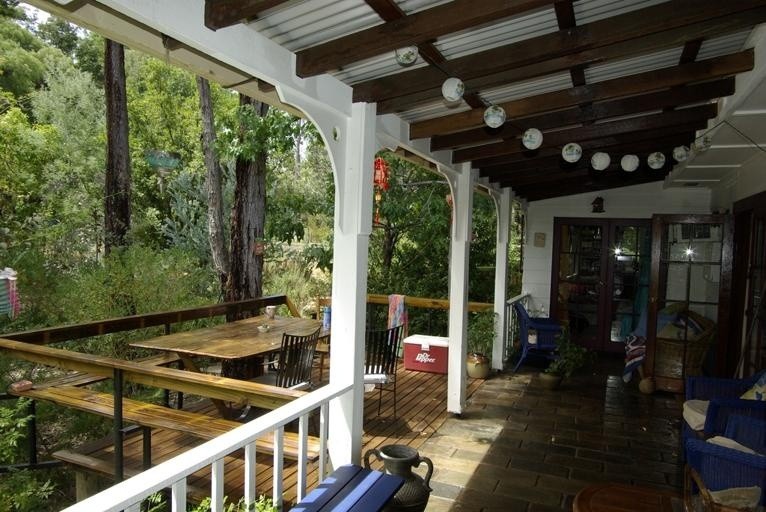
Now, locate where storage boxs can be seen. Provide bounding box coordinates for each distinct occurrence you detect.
[401,334,448,375]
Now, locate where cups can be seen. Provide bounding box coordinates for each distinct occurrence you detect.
[265,305,277,319]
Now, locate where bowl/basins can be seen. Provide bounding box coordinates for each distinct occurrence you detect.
[256,325,270,333]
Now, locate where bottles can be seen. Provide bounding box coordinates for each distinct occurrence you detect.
[323,306,331,329]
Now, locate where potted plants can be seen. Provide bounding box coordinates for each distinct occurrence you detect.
[465,311,500,378]
[539,329,588,391]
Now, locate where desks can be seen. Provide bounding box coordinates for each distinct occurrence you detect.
[128,315,330,420]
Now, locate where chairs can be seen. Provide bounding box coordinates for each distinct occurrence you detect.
[511,300,563,374]
[272,322,405,421]
[677,374,765,509]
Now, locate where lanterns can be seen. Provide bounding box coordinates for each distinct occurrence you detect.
[372,156,390,224]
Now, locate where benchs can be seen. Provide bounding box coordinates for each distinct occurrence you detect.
[285,464,407,511]
